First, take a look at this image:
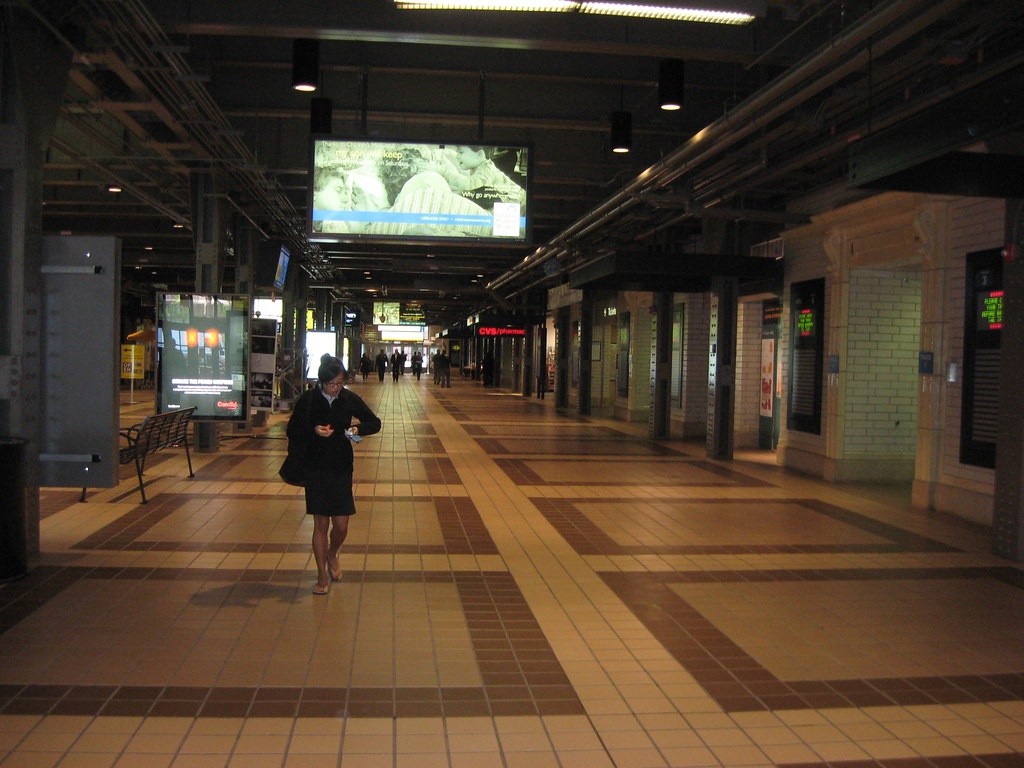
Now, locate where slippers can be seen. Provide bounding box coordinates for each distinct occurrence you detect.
[328,555,343,580]
[313,583,329,594]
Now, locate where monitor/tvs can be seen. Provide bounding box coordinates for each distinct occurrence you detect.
[307,134,536,249]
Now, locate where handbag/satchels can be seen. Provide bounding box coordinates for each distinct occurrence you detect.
[280,442,323,487]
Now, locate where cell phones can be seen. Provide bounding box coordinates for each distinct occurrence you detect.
[348,432,362,445]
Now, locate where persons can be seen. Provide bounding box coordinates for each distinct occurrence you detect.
[375,349,389,382]
[359,353,371,379]
[312,145,526,237]
[432,349,452,388]
[481,352,494,387]
[390,349,406,383]
[411,350,423,381]
[157,337,187,411]
[284,353,382,594]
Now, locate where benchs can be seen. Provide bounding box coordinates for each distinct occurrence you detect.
[79,405,198,506]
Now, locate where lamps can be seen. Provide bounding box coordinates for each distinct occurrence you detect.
[609,81,633,153]
[658,57,686,111]
[308,70,332,134]
[292,38,321,93]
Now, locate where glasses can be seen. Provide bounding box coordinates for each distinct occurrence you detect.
[325,379,344,387]
